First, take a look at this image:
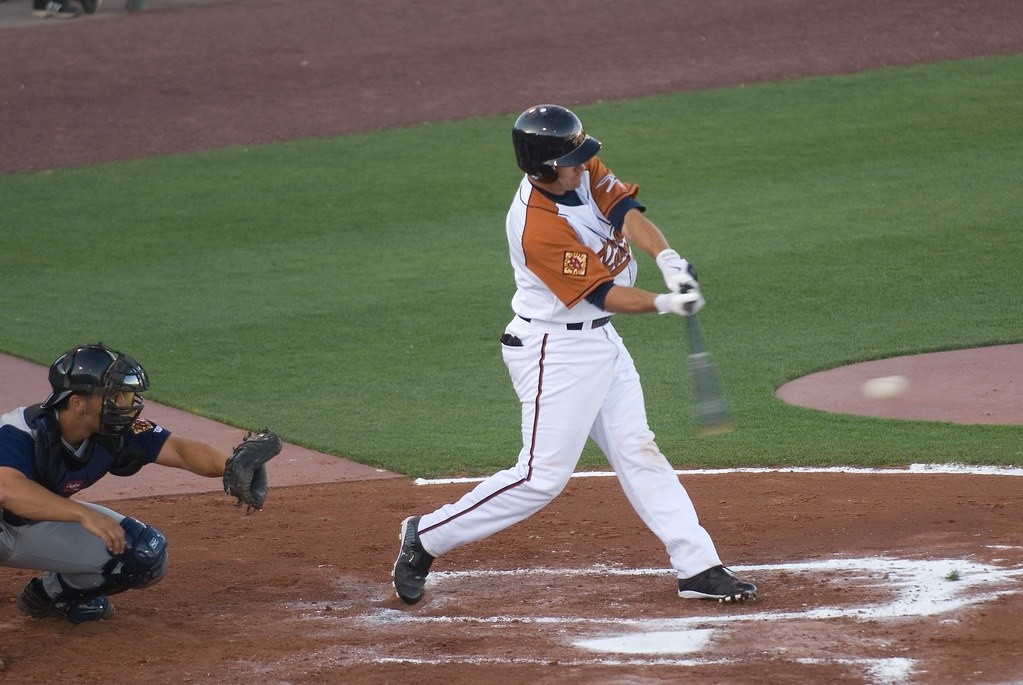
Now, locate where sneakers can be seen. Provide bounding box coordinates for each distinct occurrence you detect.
[677,565,758,603]
[17,576,108,625]
[392,516,438,602]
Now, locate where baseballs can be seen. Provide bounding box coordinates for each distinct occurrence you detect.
[865,375,907,399]
[679,287,736,439]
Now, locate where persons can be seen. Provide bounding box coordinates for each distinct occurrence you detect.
[390,103,759,602]
[33,0,101,19]
[0,341,283,623]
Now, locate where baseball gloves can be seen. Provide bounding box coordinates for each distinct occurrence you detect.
[221,428,283,512]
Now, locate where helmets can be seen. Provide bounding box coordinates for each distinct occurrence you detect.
[513,104,601,182]
[39,341,117,408]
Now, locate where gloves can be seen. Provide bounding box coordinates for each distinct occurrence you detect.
[655,291,706,316]
[656,249,698,290]
[500,333,522,346]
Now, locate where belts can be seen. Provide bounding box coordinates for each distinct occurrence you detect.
[518,313,617,330]
